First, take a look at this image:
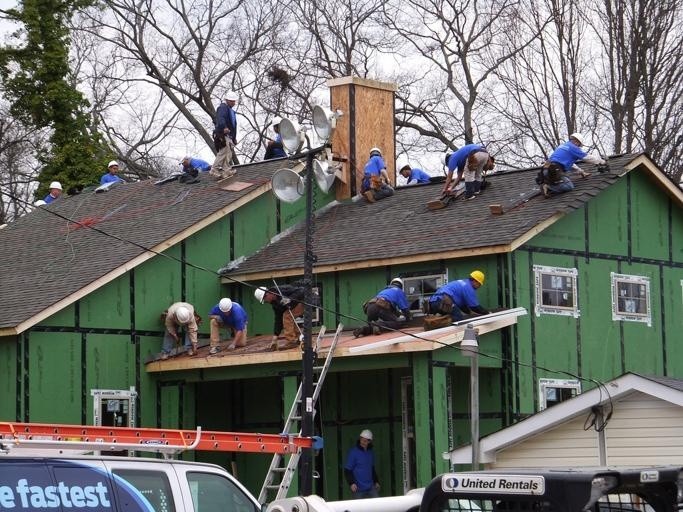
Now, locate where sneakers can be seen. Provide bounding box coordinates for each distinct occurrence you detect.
[210,168,220,177]
[185,349,193,355]
[222,170,236,178]
[540,183,550,197]
[209,346,221,354]
[473,189,480,194]
[363,189,374,202]
[369,320,379,333]
[158,352,170,360]
[353,324,365,337]
[461,193,475,201]
[283,338,299,348]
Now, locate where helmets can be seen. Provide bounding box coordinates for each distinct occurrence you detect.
[175,153,186,166]
[224,92,238,102]
[359,429,372,439]
[107,160,118,168]
[439,152,449,166]
[569,133,585,146]
[470,270,484,286]
[253,287,268,304]
[396,162,409,174]
[370,147,381,156]
[218,298,231,312]
[388,278,404,290]
[175,306,188,324]
[48,181,62,191]
[272,116,282,126]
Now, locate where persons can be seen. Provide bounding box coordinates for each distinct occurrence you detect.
[160,302,199,360]
[344,427,382,500]
[398,160,432,185]
[99,161,125,185]
[207,296,249,354]
[178,155,212,172]
[352,278,415,340]
[360,148,394,203]
[536,132,611,198]
[263,123,284,160]
[210,90,239,178]
[440,143,491,201]
[42,181,63,204]
[421,270,492,332]
[253,282,320,352]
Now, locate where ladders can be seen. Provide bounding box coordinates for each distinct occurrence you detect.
[0,422,324,454]
[257,322,345,505]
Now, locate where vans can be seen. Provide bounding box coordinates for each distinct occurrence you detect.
[2,450,267,509]
